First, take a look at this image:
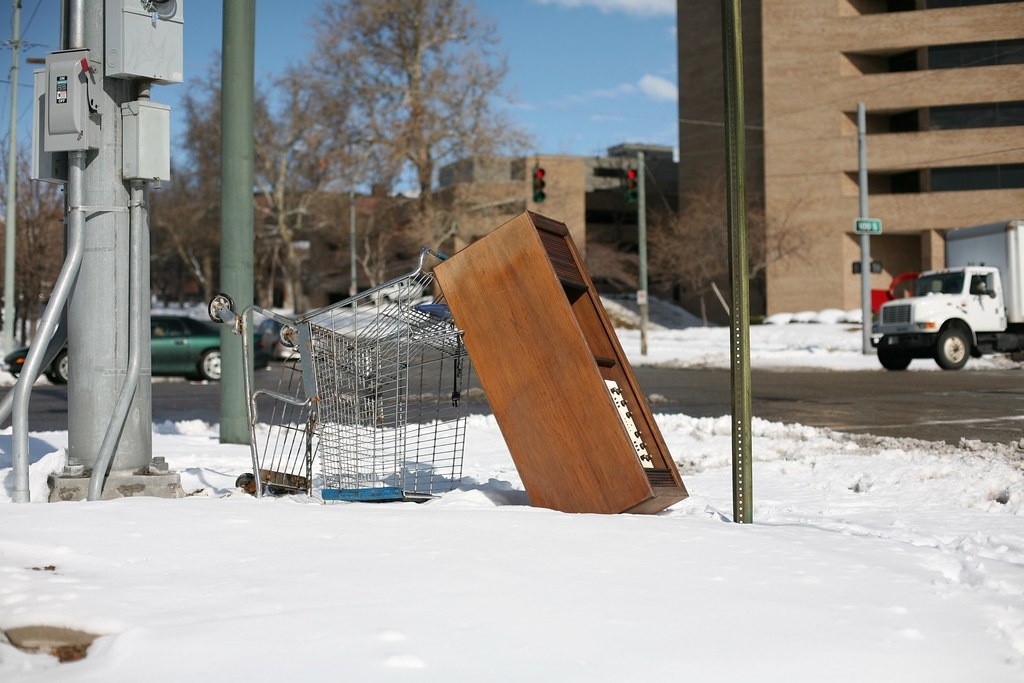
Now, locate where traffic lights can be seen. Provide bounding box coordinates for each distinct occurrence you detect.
[532,167,546,203]
[624,169,638,205]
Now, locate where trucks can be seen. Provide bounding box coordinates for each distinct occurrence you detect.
[869,217,1024,374]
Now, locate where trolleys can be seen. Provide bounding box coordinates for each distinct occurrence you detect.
[208,246,472,505]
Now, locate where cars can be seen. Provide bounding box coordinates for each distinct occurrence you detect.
[2,314,269,387]
[256,318,301,362]
